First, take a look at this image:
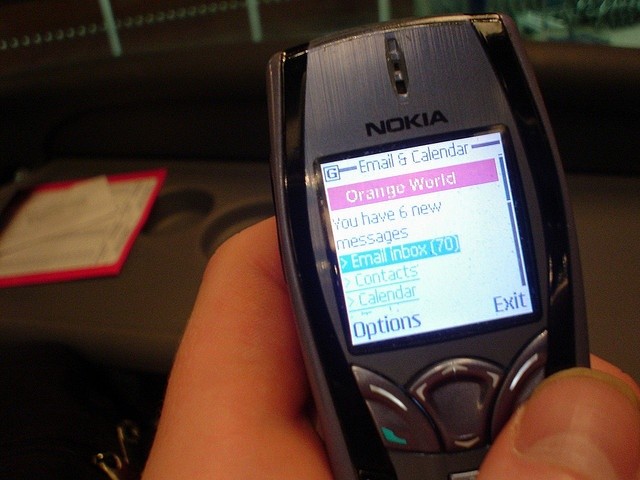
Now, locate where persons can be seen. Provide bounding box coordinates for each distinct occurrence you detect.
[140,215,640,479]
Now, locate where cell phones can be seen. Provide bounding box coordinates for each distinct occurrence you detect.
[265,12,591,479]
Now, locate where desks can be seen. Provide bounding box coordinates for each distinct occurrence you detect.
[1,156,638,414]
